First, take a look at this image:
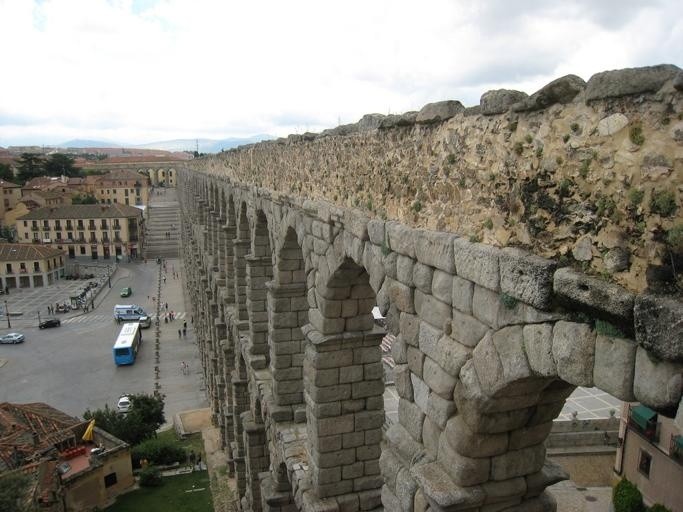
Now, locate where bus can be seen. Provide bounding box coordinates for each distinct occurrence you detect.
[113,323,142,366]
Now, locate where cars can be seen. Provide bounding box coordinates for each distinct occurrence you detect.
[39,319,61,329]
[139,315,151,328]
[0,332,24,344]
[117,394,135,413]
[121,287,132,297]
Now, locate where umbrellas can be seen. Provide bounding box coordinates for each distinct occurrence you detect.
[82,417,99,441]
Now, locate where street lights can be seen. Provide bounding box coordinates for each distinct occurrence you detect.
[107,264,111,288]
[4,301,11,328]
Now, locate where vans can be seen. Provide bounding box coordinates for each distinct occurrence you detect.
[114,304,147,321]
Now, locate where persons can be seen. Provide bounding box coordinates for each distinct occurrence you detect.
[181,361,190,375]
[47,301,72,315]
[5,286,9,296]
[189,450,202,473]
[144,255,194,339]
[165,224,177,239]
